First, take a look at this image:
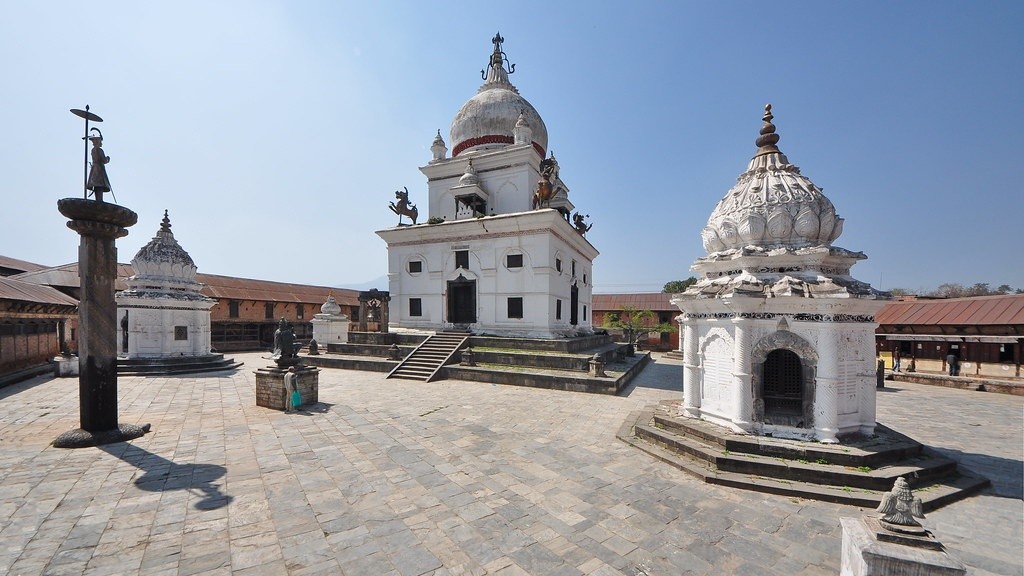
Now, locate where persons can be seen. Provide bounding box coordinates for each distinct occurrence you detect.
[284,366,299,413]
[892,346,901,372]
[906,356,916,372]
[947,350,959,376]
[87,136,110,202]
[273,316,302,358]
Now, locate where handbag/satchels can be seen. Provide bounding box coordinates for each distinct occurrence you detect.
[292,391,301,406]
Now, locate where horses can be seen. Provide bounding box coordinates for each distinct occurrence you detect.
[533,166,561,209]
[574,215,593,235]
[389,191,418,224]
